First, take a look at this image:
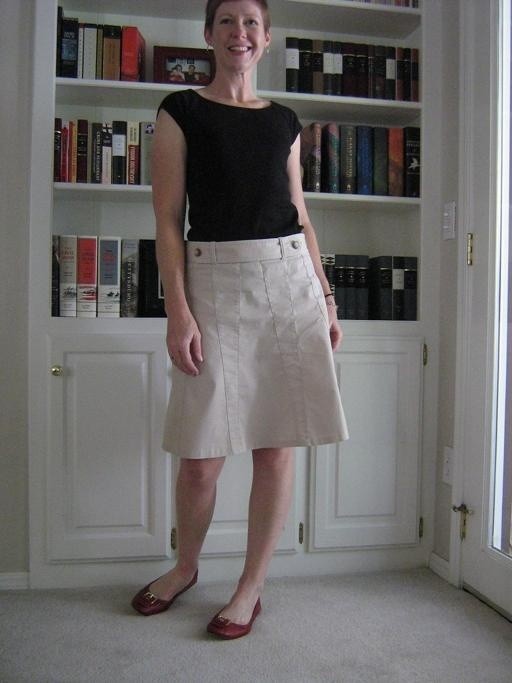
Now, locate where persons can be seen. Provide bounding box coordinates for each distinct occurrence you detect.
[132,0,343,639]
[167,65,200,83]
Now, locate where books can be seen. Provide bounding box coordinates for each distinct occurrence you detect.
[302,121,418,195]
[57,8,147,78]
[52,234,169,316]
[54,116,153,184]
[318,253,417,318]
[286,36,418,100]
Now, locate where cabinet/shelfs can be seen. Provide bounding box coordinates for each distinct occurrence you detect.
[24,0,442,588]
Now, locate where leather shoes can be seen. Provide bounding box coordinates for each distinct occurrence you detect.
[129,567,200,618]
[204,593,263,641]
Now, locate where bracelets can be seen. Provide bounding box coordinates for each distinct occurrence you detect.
[323,292,337,308]
